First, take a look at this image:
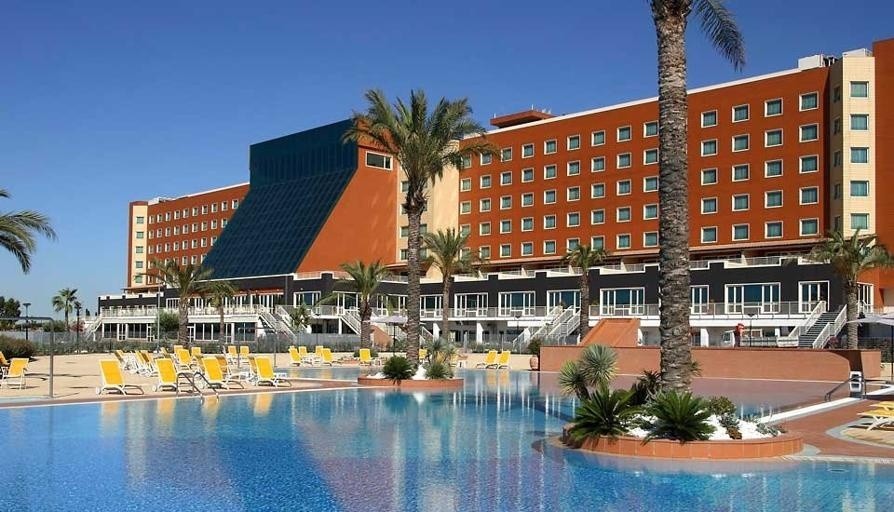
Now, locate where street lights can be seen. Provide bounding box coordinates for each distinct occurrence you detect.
[23,301,32,340]
[746,312,755,347]
[156,284,168,353]
[514,314,522,354]
[74,304,82,354]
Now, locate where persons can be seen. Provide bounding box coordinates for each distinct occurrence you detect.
[734,323,742,347]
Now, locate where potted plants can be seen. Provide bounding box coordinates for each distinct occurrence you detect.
[526,337,543,370]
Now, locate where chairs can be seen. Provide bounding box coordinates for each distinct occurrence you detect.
[0,357,29,391]
[869,400,894,411]
[856,409,894,432]
[0,351,10,379]
